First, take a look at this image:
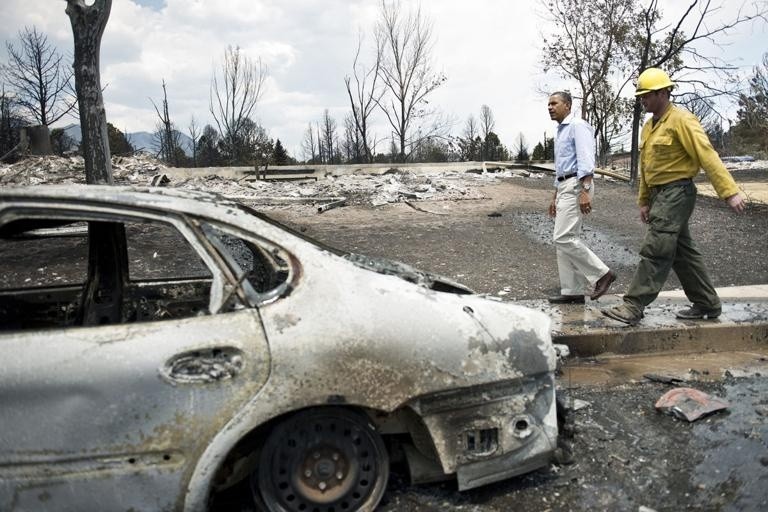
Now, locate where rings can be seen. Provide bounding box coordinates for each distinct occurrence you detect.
[586,206,589,210]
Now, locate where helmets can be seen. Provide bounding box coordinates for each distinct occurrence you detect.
[635,68,673,96]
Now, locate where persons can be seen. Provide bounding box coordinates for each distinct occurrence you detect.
[600,67,749,325]
[547,92,617,302]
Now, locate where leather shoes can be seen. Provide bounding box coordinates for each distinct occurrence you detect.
[547,295,584,303]
[591,269,617,299]
[601,305,640,324]
[675,304,720,319]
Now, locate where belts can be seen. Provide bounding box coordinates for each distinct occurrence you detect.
[651,179,689,192]
[558,173,577,181]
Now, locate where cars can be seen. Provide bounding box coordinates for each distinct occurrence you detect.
[0,181,560,512]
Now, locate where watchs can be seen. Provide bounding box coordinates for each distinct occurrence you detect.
[581,184,592,190]
